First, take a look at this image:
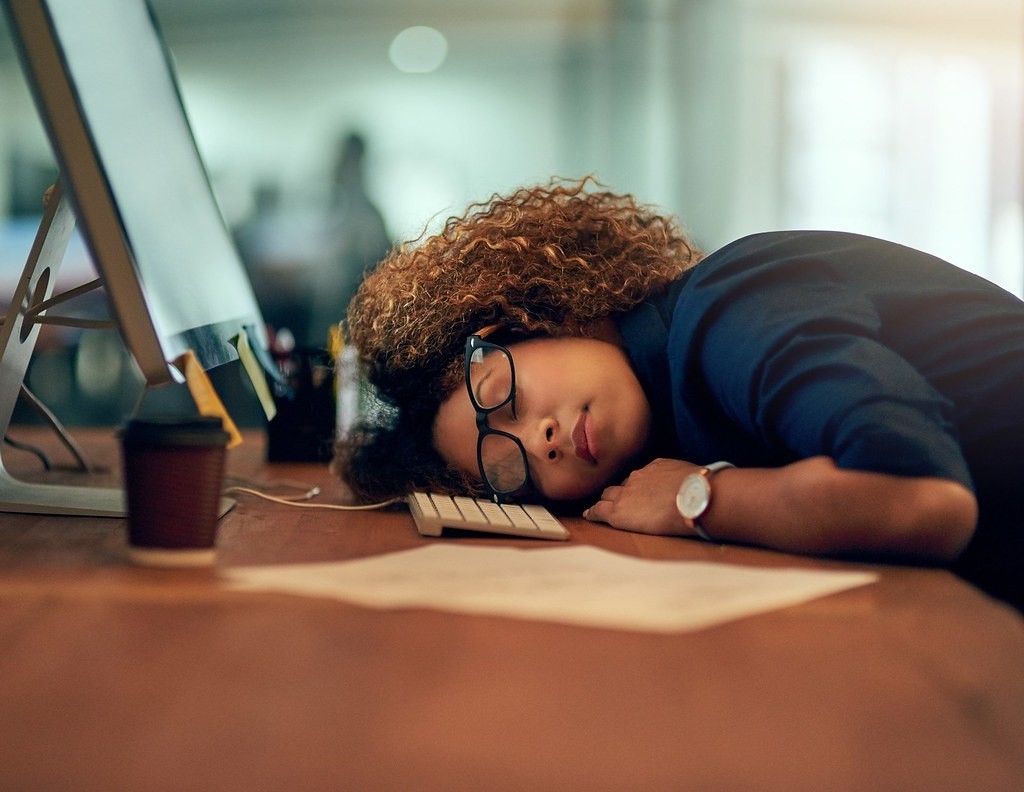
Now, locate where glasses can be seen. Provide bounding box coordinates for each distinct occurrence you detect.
[465,318,530,505]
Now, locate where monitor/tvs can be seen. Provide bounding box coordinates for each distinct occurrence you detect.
[0,0,270,522]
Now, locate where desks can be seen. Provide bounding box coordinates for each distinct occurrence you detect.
[0,425,1024,792]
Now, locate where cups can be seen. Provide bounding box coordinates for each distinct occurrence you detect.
[120,415,231,567]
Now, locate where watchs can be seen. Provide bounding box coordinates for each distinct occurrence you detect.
[674,460,736,545]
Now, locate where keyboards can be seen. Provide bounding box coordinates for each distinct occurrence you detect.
[407,492,570,541]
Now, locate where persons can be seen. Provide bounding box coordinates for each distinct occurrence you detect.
[330,174,1024,625]
